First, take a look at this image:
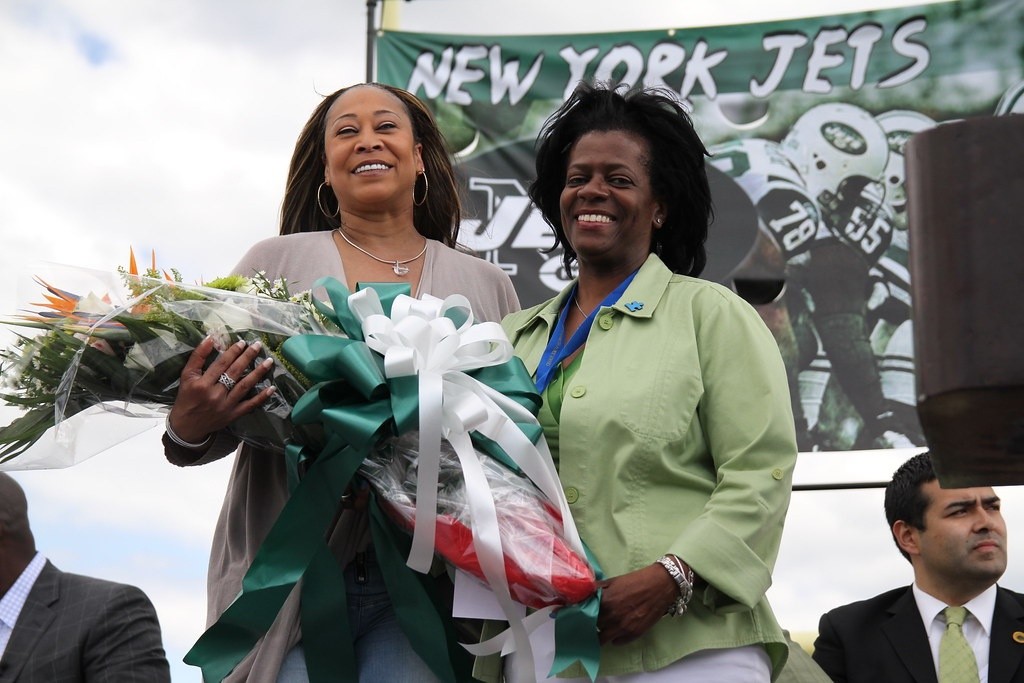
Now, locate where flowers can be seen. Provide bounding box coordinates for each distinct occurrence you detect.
[0,245,604,683]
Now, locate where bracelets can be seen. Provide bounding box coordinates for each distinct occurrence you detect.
[656,553,694,618]
[165,413,212,449]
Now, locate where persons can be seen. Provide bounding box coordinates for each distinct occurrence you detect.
[442,69,1024,454]
[162,82,521,683]
[470,80,800,683]
[812,449,1024,683]
[0,470,171,683]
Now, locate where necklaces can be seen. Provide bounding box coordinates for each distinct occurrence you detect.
[573,295,588,318]
[338,228,428,275]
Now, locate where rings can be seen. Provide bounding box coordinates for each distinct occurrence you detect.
[218,373,236,391]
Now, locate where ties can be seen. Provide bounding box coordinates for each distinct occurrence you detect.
[937,605,979,683]
